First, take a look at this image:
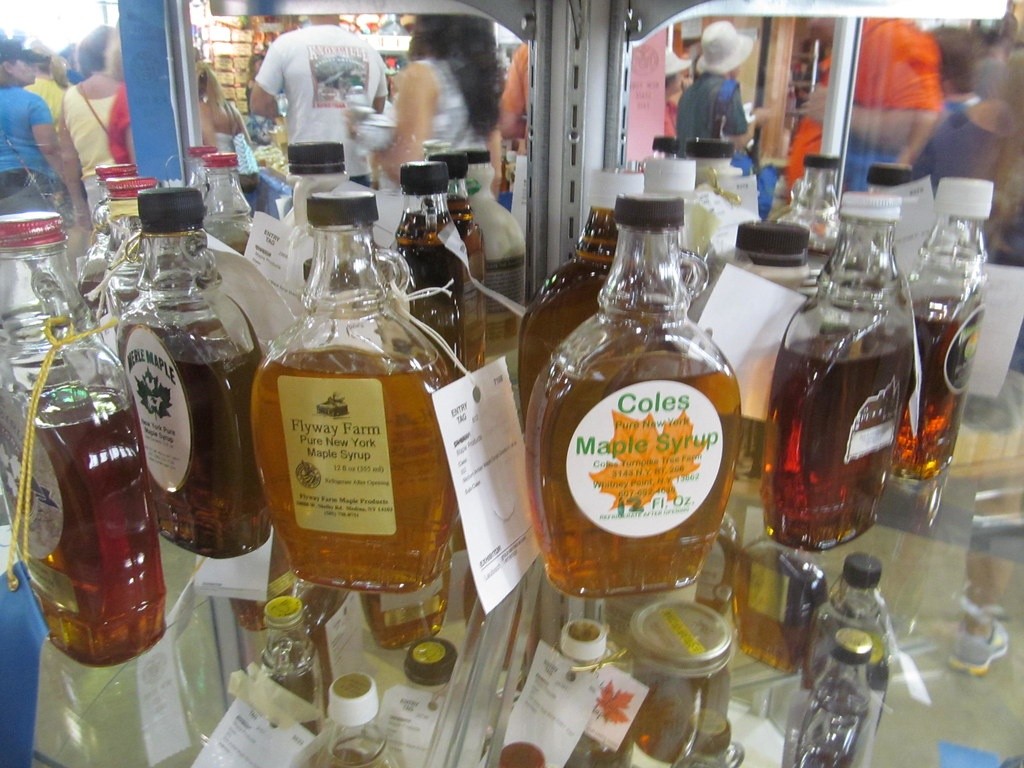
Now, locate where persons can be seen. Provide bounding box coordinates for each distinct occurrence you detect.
[663,12,1024,384]
[0,15,528,276]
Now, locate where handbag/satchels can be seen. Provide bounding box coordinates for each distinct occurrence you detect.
[718,78,777,223]
[223,101,260,193]
[50,198,75,232]
[510,117,529,242]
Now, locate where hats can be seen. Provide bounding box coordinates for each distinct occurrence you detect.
[664,46,691,78]
[0,37,40,63]
[695,20,753,74]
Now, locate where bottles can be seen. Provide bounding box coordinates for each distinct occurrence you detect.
[793,627,872,768]
[525,194,743,599]
[117,188,271,559]
[187,147,254,257]
[429,153,486,381]
[315,672,400,768]
[891,178,995,482]
[560,619,632,768]
[499,742,547,768]
[234,547,458,693]
[103,177,157,359]
[604,513,826,768]
[76,163,138,319]
[643,135,843,499]
[250,191,460,593]
[518,172,645,430]
[802,551,891,689]
[261,596,326,768]
[389,161,464,383]
[0,212,166,667]
[422,140,450,160]
[762,165,916,553]
[288,142,396,297]
[465,150,525,383]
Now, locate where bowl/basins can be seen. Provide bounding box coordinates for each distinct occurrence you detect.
[357,123,396,151]
